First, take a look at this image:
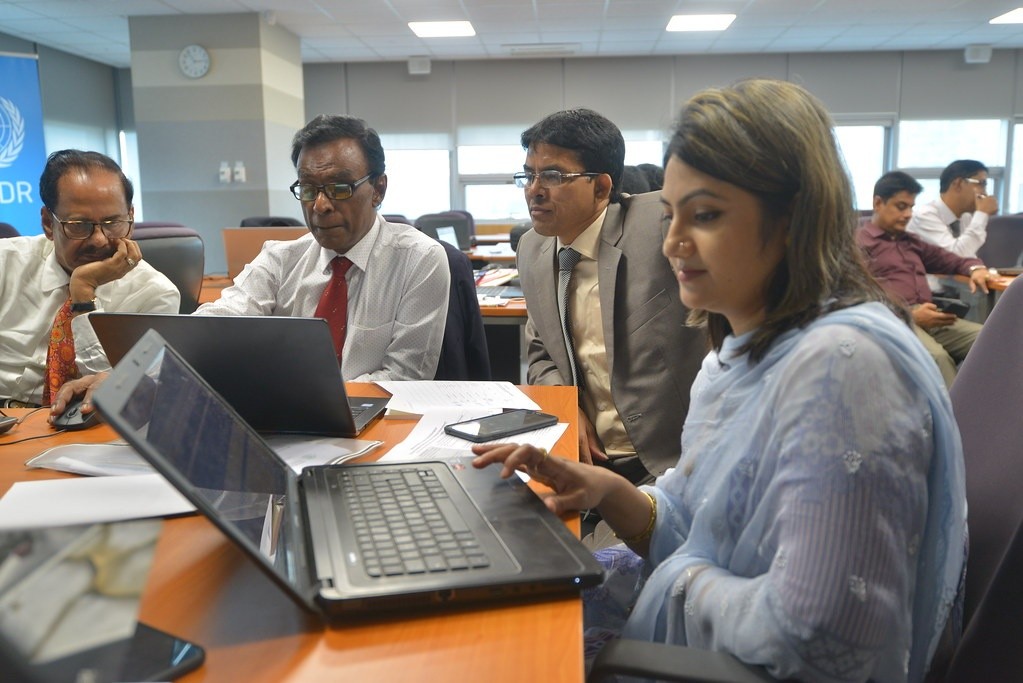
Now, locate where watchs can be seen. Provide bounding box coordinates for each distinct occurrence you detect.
[968,264,986,271]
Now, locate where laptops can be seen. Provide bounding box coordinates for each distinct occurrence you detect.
[88,313,390,439]
[89,325,606,623]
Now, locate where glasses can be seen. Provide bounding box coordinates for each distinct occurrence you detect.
[514,169,616,189]
[46,207,133,240]
[290,170,375,202]
[962,175,987,190]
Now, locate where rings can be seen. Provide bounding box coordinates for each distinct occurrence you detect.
[126,257,135,266]
[534,447,547,475]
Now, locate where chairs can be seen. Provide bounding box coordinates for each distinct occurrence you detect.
[0,168,1023,682]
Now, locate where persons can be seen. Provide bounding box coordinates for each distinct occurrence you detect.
[905,159,999,309]
[852,169,985,392]
[0,149,181,409]
[50,114,451,415]
[470,77,970,683]
[516,107,712,553]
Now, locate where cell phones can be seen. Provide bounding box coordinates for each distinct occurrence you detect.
[942,302,962,313]
[444,409,559,443]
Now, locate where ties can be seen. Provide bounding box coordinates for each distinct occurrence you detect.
[949,220,960,238]
[313,257,354,373]
[40,291,79,408]
[556,248,581,405]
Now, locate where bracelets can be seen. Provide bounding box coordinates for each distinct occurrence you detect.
[70,301,96,312]
[614,493,656,544]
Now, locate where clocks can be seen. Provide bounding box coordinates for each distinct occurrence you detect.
[179,43,213,77]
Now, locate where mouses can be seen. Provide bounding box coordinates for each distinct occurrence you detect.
[53,399,104,431]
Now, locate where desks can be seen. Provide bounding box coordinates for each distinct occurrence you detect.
[0,380,583,680]
[464,240,516,268]
[924,265,1016,324]
[474,282,532,385]
